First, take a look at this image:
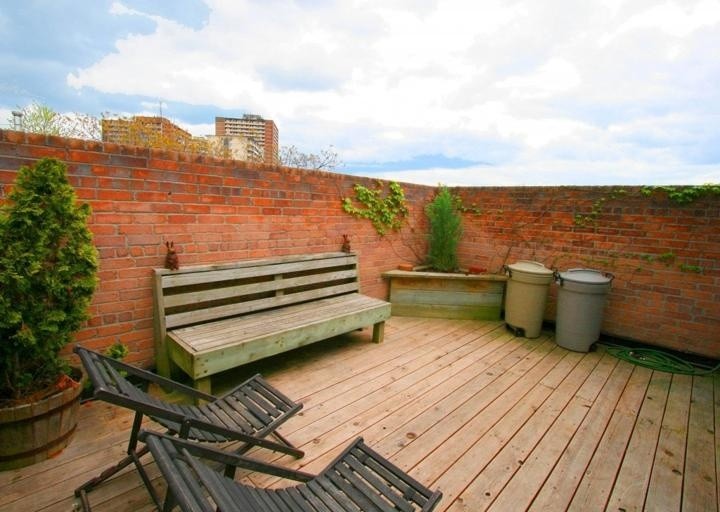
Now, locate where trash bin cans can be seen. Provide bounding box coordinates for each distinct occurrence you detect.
[503,260,615,352]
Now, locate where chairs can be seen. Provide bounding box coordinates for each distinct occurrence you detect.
[135,426,443,512]
[70,344,305,512]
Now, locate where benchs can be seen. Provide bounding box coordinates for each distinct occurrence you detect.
[155,250,392,406]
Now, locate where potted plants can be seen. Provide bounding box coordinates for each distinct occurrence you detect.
[0,151,98,473]
[383,183,507,321]
